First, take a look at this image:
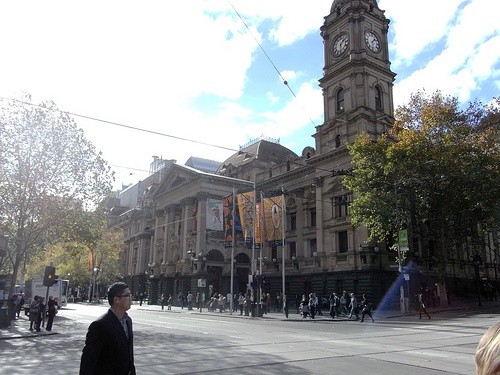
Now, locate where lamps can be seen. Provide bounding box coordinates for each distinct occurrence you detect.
[292,255,299,269]
[374,244,379,255]
[312,249,321,267]
[273,258,279,272]
[358,244,363,256]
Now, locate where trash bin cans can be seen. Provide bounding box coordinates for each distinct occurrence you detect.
[400,297,409,315]
[250,302,267,318]
[0,307,9,329]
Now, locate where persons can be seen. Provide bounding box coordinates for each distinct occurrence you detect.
[12,292,25,317]
[139,291,283,324]
[45,295,57,331]
[80,282,137,375]
[28,296,46,332]
[329,291,375,323]
[419,294,431,320]
[295,293,323,319]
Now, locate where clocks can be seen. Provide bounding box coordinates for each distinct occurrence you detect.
[365,31,380,53]
[332,34,348,56]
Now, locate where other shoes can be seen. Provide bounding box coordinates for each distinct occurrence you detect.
[372,320,374,322]
[360,321,364,323]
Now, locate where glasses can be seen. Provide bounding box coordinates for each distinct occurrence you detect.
[117,294,132,300]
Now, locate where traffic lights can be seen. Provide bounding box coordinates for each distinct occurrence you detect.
[53,275,59,284]
[43,266,55,286]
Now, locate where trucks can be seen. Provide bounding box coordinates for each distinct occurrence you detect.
[15,279,69,315]
